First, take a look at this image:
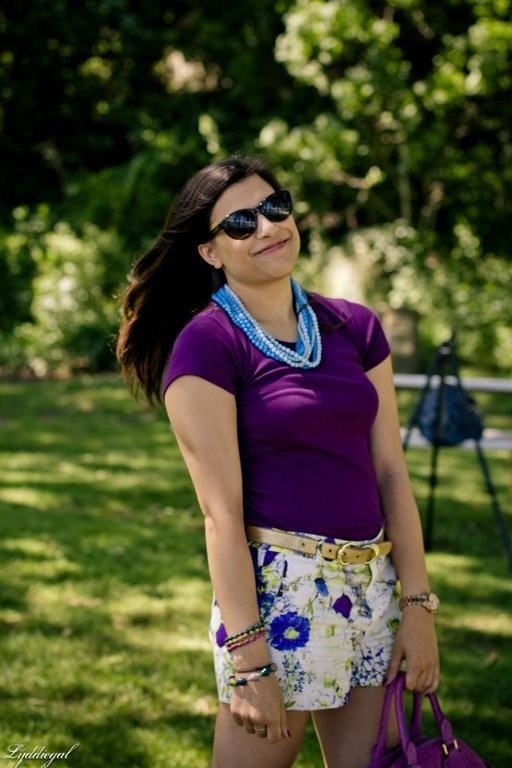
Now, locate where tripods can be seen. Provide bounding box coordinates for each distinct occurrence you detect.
[402,374,512,553]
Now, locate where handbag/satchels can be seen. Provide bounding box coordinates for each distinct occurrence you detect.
[367,670,487,768]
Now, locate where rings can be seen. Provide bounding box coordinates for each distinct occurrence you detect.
[255,727,265,732]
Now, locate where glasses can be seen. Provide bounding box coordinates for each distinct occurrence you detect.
[203,188,297,243]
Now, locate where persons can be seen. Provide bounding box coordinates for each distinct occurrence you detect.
[110,158,444,767]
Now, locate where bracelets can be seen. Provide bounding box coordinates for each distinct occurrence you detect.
[225,662,279,687]
[398,591,440,614]
[224,622,269,652]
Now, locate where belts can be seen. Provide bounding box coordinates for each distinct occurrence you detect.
[242,515,396,567]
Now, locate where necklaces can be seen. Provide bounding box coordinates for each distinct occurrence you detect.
[211,273,324,370]
